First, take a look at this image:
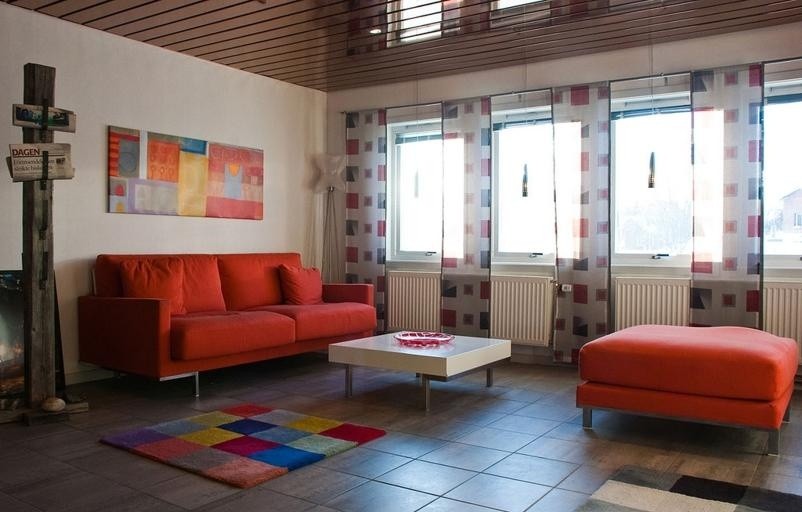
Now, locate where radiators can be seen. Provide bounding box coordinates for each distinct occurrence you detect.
[614,277,802,366]
[387,270,552,348]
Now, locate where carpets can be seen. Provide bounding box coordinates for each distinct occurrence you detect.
[101,403,385,490]
[572,465,801,512]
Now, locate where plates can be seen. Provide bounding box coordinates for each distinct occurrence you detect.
[396,331,453,350]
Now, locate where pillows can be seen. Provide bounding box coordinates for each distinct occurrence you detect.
[278,265,323,305]
[122,257,186,314]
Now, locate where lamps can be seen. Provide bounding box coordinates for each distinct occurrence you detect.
[315,154,345,284]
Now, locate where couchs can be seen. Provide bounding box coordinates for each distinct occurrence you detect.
[80,253,376,396]
[576,325,800,456]
